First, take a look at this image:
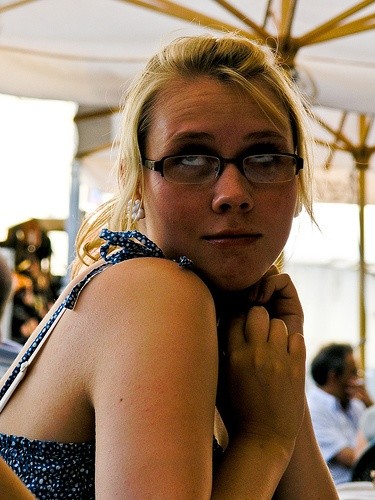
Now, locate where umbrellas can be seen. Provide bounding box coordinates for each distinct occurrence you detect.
[73,101,375,379]
[1,0,375,114]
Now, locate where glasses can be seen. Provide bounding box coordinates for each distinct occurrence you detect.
[138,154,304,185]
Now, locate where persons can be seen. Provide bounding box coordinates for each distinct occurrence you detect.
[0,34,341,500]
[305,342,375,488]
